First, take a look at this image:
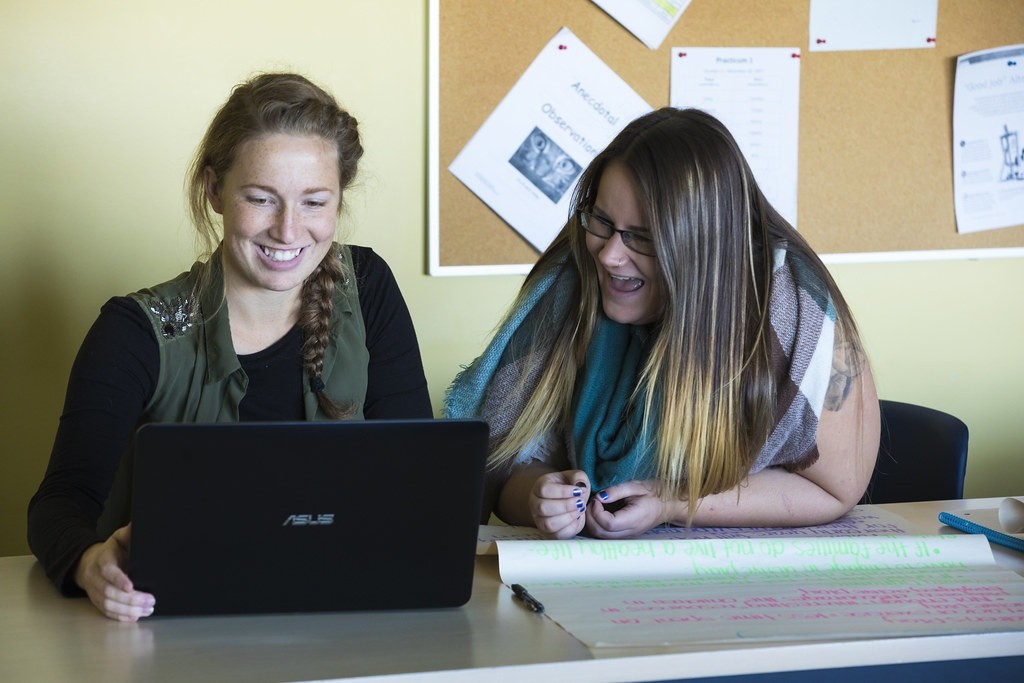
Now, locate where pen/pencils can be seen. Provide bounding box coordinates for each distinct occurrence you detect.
[511,583,545,613]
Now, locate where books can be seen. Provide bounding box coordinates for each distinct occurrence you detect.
[938,508,1024,554]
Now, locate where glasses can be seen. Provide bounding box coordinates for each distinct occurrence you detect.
[576,197,658,257]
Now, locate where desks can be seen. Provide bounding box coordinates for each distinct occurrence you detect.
[0,495,1024,683]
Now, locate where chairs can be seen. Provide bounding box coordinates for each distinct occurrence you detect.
[859,401,970,504]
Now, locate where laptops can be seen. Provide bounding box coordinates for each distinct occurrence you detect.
[123,420,492,621]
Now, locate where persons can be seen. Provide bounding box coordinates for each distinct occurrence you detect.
[27,75,434,623]
[444,107,880,540]
